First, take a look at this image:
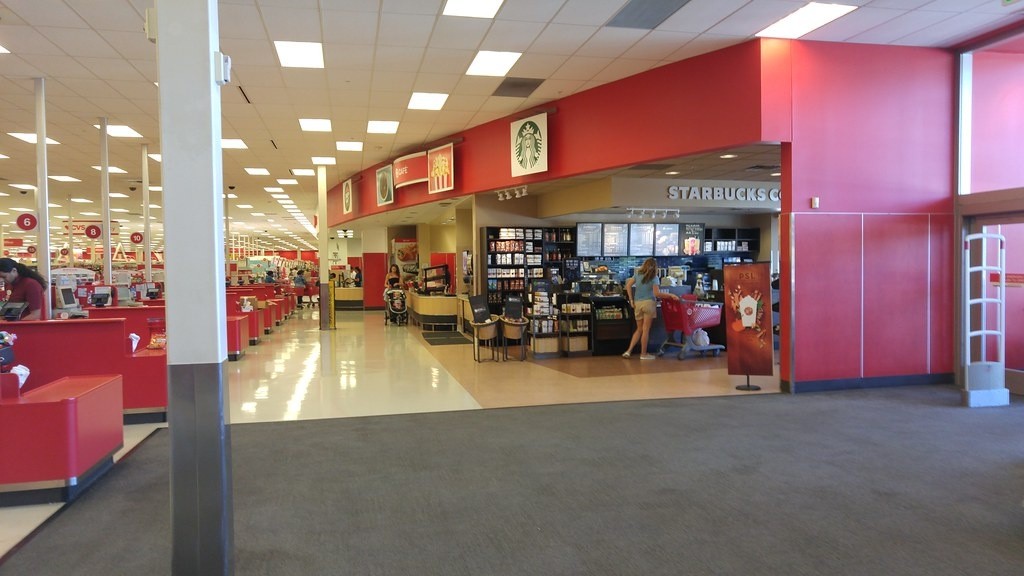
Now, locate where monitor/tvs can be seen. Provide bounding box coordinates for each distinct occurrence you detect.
[55,286,78,309]
[668,267,687,282]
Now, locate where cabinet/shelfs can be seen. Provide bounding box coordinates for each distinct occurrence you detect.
[480,226,596,346]
[561,313,595,358]
[525,310,563,358]
[704,227,761,272]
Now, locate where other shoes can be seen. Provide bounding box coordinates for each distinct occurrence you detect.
[622,351,631,359]
[639,352,655,359]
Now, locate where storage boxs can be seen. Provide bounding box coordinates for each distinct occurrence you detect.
[485,228,591,334]
[717,241,754,270]
[704,242,713,252]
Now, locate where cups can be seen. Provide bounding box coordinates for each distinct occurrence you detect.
[740,302,757,327]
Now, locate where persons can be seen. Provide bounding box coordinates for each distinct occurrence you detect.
[348,266,357,287]
[293,270,307,307]
[622,258,680,359]
[329,272,336,280]
[0,258,49,320]
[354,267,362,287]
[287,267,318,280]
[265,271,276,283]
[384,263,405,322]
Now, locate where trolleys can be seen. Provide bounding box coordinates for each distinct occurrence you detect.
[656,295,726,360]
[303,281,319,308]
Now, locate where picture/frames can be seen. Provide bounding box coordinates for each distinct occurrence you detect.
[575,222,706,258]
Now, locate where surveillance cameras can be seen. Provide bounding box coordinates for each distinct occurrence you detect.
[229,186,235,190]
[20,191,27,194]
[130,187,136,192]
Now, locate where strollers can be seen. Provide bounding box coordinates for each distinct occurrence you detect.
[383,284,409,326]
